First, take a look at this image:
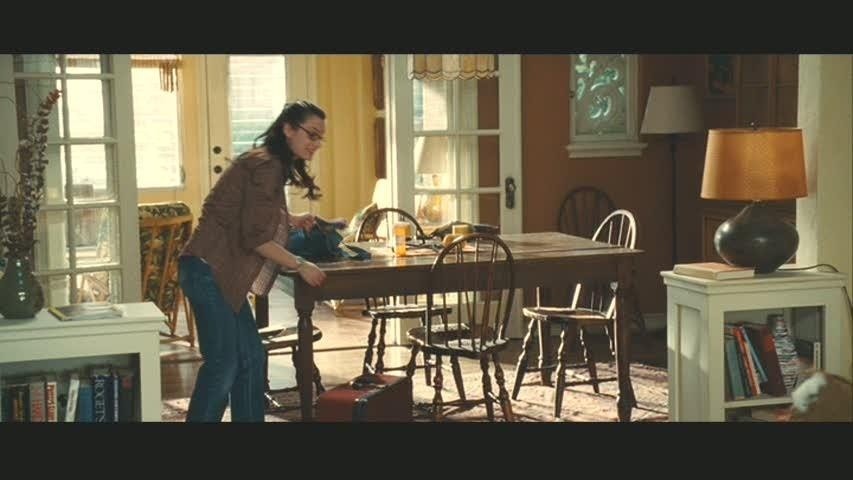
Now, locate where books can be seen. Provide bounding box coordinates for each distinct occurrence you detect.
[723,313,821,403]
[0,360,141,422]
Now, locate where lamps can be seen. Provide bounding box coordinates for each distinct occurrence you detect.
[700,120,810,274]
[640,85,706,264]
[417,135,447,222]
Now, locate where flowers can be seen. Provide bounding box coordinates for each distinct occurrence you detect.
[0,86,61,249]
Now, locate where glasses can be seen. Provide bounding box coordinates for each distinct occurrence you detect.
[299,125,322,141]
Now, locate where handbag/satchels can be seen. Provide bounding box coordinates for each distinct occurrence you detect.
[287,216,343,262]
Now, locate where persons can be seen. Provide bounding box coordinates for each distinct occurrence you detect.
[172,95,333,423]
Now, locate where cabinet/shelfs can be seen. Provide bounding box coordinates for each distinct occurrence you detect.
[660,265,853,422]
[0,301,166,422]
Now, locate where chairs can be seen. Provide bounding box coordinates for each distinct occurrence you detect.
[407,232,516,422]
[256,326,325,411]
[557,187,623,339]
[138,202,195,346]
[513,210,646,418]
[355,208,434,387]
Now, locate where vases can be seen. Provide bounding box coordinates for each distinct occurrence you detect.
[0,253,41,319]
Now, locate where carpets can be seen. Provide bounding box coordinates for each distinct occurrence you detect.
[159,361,668,423]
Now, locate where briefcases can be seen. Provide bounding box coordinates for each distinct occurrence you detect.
[317,373,412,422]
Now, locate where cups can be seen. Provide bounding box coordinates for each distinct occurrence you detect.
[394,223,406,258]
[452,224,469,248]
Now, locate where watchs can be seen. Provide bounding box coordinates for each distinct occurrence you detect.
[293,256,304,272]
